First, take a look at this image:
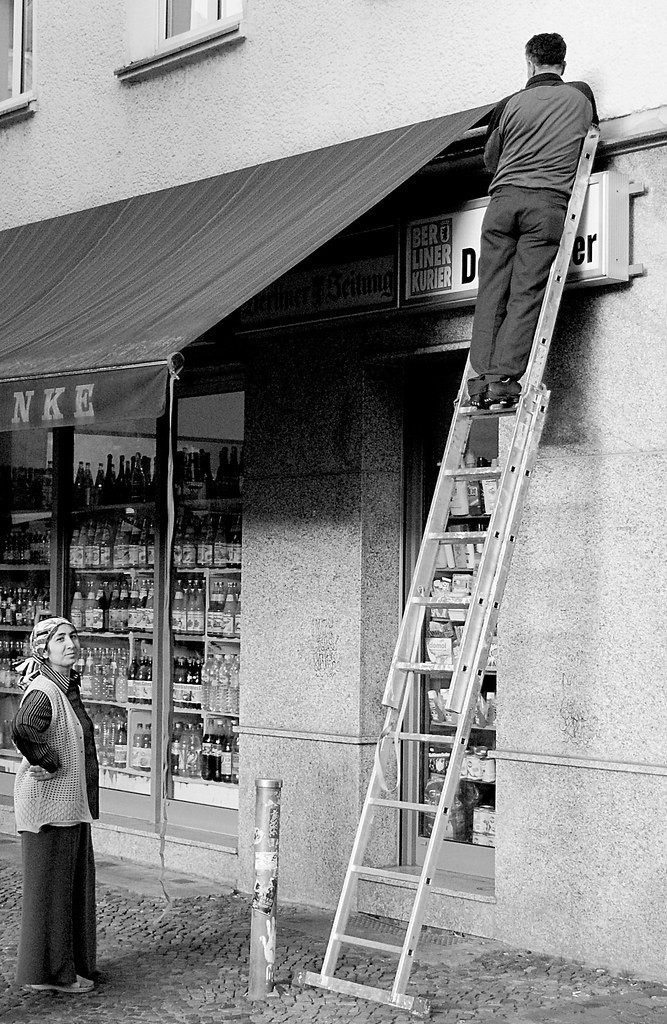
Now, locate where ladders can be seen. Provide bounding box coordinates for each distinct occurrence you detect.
[294,124,601,1014]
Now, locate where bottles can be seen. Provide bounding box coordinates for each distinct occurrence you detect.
[0,575,241,785]
[2,446,245,569]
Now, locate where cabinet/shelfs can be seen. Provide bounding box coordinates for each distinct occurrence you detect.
[414,514,494,879]
[0,497,243,810]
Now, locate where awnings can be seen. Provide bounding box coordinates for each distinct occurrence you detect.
[0,101,500,432]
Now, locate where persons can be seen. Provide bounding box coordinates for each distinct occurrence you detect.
[468,33,599,408]
[9,616,100,993]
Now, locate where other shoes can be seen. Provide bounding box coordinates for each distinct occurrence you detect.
[470,399,490,410]
[31,974,94,992]
[489,394,521,410]
